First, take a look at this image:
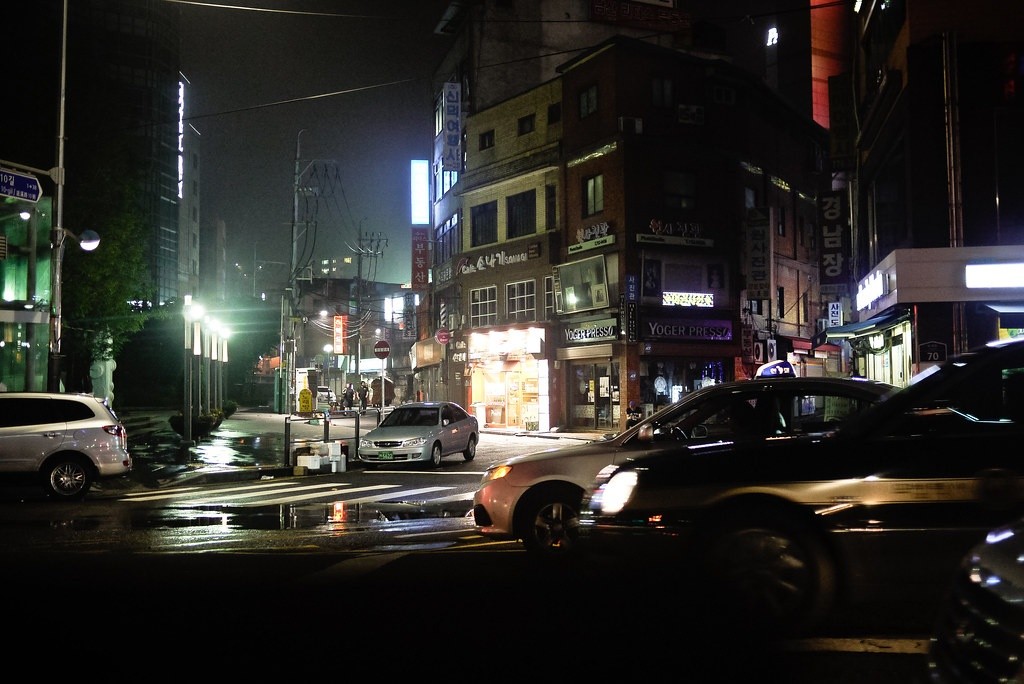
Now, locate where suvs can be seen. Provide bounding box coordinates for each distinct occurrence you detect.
[0,391,132,505]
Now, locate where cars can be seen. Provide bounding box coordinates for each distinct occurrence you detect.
[561,335,1024,646]
[357,400,479,470]
[925,520,1024,684]
[471,375,902,560]
[317,385,336,403]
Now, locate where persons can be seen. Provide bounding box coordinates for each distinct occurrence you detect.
[342,383,355,416]
[357,381,369,417]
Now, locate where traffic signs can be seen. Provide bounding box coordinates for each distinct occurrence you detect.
[0,169,43,203]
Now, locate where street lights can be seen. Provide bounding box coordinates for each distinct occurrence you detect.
[323,344,333,404]
[180,294,233,448]
[52,227,101,392]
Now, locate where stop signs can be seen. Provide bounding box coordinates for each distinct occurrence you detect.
[374,339,390,360]
